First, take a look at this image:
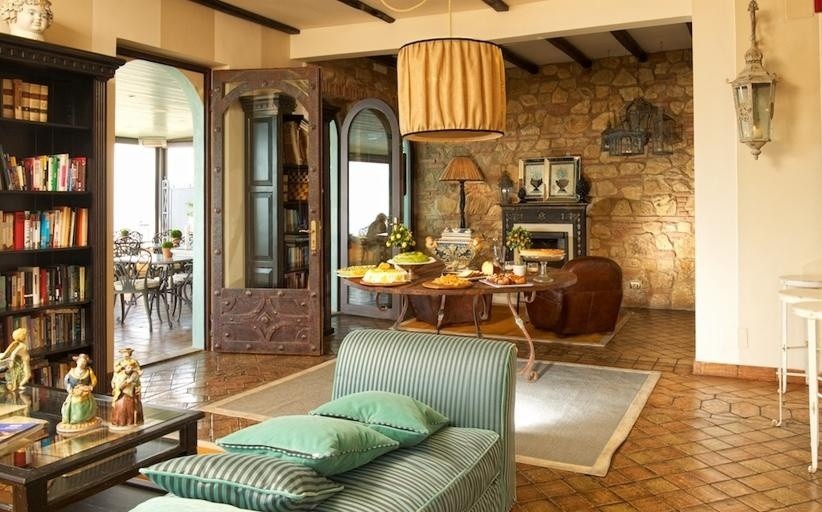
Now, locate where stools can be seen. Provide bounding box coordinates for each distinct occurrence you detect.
[770,271,822,478]
[410,263,494,324]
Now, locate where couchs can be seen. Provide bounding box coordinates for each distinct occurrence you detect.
[131,328,520,512]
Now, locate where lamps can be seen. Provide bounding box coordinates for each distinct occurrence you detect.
[730,0,779,158]
[435,154,489,236]
[391,0,511,145]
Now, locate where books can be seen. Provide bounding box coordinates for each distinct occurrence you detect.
[1,78,48,123]
[284,118,310,289]
[0,151,90,466]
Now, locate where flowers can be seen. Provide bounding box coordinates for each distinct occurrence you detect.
[384,219,414,253]
[503,226,531,253]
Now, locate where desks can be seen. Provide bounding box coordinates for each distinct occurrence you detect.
[338,259,581,386]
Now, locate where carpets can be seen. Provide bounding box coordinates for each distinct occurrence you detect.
[195,353,666,479]
[399,305,636,350]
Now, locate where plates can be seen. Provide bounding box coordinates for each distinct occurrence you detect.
[455,274,490,281]
[359,277,408,287]
[336,263,376,279]
[480,279,534,288]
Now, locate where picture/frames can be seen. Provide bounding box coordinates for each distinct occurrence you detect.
[542,156,582,204]
[517,154,543,201]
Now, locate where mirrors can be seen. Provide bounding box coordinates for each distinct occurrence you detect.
[346,107,391,313]
[221,76,310,289]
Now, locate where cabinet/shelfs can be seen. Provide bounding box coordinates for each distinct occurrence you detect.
[0,31,126,397]
[237,90,312,291]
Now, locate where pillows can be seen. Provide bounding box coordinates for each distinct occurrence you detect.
[304,387,453,450]
[137,453,345,511]
[212,413,400,476]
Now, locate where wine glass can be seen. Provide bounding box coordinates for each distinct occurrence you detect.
[523,254,564,283]
[388,256,436,281]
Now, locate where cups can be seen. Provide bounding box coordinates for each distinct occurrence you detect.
[505,261,539,277]
[434,228,476,274]
[494,246,507,273]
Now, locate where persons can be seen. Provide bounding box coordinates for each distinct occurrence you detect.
[112,365,144,426]
[111,347,142,405]
[62,353,98,423]
[365,212,388,267]
[1,0,53,41]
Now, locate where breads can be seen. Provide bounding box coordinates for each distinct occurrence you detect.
[520,248,566,256]
[488,272,527,285]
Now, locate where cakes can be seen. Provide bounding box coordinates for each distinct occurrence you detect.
[362,261,408,283]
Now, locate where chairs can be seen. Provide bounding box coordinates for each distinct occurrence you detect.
[525,254,625,338]
[108,225,192,333]
